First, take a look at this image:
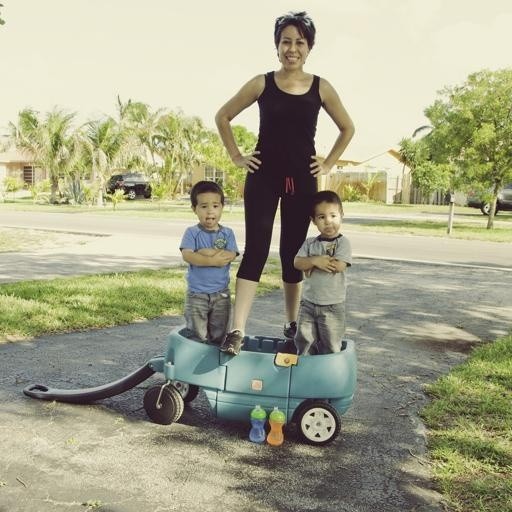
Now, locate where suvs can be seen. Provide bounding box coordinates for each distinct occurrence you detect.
[109,174,151,199]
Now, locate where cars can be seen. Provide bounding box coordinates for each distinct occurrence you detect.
[467,179,511,215]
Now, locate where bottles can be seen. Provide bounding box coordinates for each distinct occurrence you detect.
[249,405,286,446]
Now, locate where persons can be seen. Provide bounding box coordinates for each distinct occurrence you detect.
[291,189,354,360]
[174,179,242,343]
[213,11,355,358]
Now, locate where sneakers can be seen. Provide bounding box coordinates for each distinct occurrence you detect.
[219,328,245,356]
[283,321,298,339]
